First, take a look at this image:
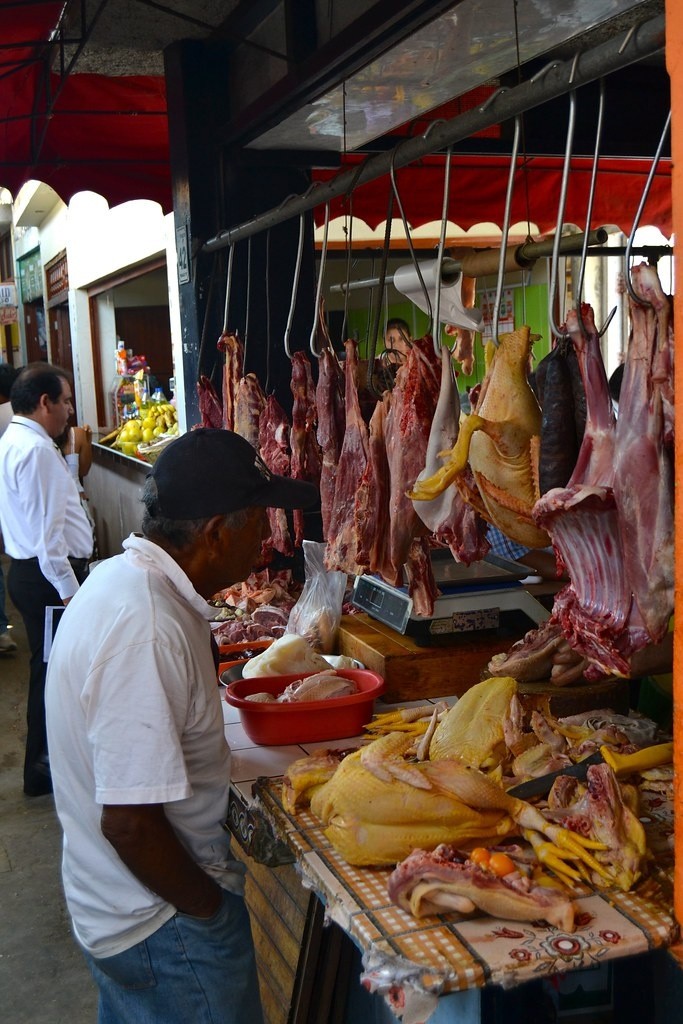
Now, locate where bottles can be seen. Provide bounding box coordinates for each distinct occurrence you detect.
[151,388,166,405]
[142,389,151,405]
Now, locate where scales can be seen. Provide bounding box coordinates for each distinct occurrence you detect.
[348,549,554,649]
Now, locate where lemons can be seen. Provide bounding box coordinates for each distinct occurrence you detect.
[117,418,165,454]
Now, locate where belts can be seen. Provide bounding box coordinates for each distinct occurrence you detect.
[68,558,91,573]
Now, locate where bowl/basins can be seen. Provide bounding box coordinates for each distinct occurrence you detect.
[219,654,365,687]
[224,669,386,745]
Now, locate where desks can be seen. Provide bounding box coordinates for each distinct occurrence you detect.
[247,774,683,1024]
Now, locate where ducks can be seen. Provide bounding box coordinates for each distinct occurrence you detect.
[387,843,579,934]
[361,677,592,779]
[538,761,654,891]
[243,670,359,703]
[402,319,552,550]
[281,731,614,891]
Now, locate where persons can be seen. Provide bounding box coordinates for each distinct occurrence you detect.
[0,362,94,796]
[484,520,565,582]
[550,631,675,685]
[52,416,93,499]
[44,428,320,1024]
[375,319,412,366]
[0,363,17,652]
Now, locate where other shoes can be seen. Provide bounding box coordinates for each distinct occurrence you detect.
[0,634,18,651]
[23,761,53,797]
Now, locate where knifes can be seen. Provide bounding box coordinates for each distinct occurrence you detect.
[505,743,674,801]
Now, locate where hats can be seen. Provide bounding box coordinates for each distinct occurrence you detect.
[139,428,321,518]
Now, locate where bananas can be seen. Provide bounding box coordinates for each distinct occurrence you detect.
[149,405,177,435]
[99,427,122,448]
